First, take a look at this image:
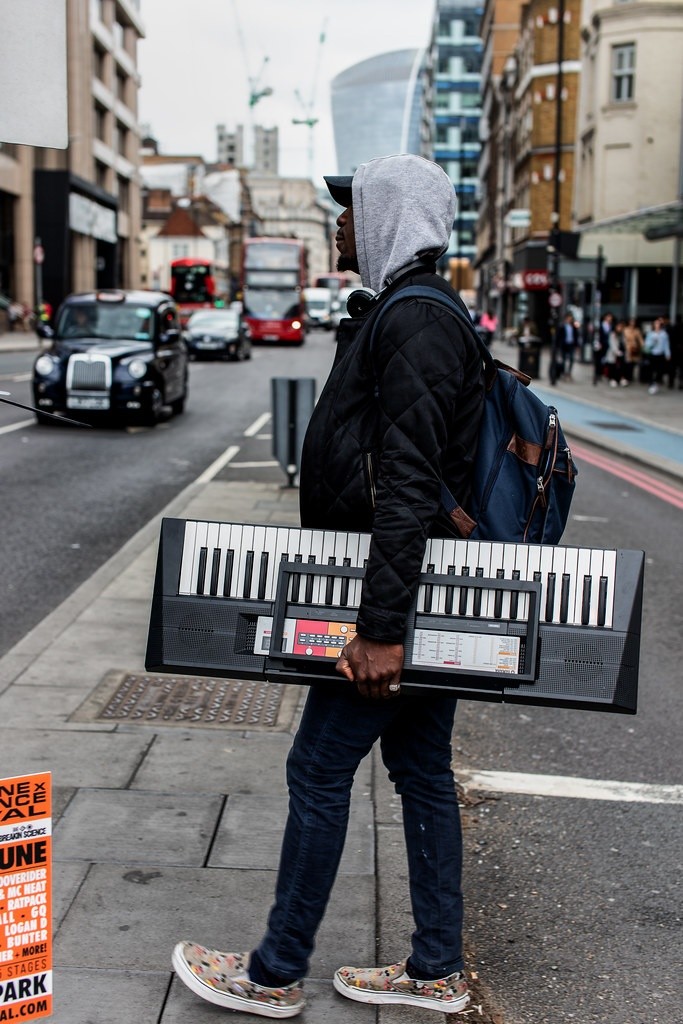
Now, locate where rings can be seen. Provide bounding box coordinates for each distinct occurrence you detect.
[389,684,400,691]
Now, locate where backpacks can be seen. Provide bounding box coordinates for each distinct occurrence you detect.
[366,286,579,546]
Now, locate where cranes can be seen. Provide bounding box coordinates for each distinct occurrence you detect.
[230,1,329,176]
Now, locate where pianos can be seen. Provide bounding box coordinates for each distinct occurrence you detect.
[142,514,648,718]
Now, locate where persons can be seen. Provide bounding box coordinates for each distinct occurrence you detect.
[6,296,51,333]
[171,154,485,1019]
[468,306,683,395]
[63,309,96,338]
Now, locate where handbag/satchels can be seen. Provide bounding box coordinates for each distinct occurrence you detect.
[631,348,640,356]
[549,355,564,379]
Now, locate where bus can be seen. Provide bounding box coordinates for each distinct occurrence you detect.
[170,257,233,324]
[241,238,310,346]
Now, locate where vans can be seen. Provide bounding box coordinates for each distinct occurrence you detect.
[332,289,352,326]
[311,274,357,290]
[305,288,335,331]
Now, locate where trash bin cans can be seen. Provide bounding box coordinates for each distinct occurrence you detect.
[270,376,316,490]
[518,337,543,380]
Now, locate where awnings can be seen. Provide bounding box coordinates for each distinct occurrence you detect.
[572,199,683,241]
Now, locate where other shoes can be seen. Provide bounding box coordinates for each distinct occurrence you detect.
[171,940,305,1018]
[609,378,629,388]
[333,957,470,1013]
[648,384,683,395]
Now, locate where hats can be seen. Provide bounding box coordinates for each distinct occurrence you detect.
[323,176,353,208]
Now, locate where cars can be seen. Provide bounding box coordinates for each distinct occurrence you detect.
[30,289,193,428]
[182,307,251,362]
